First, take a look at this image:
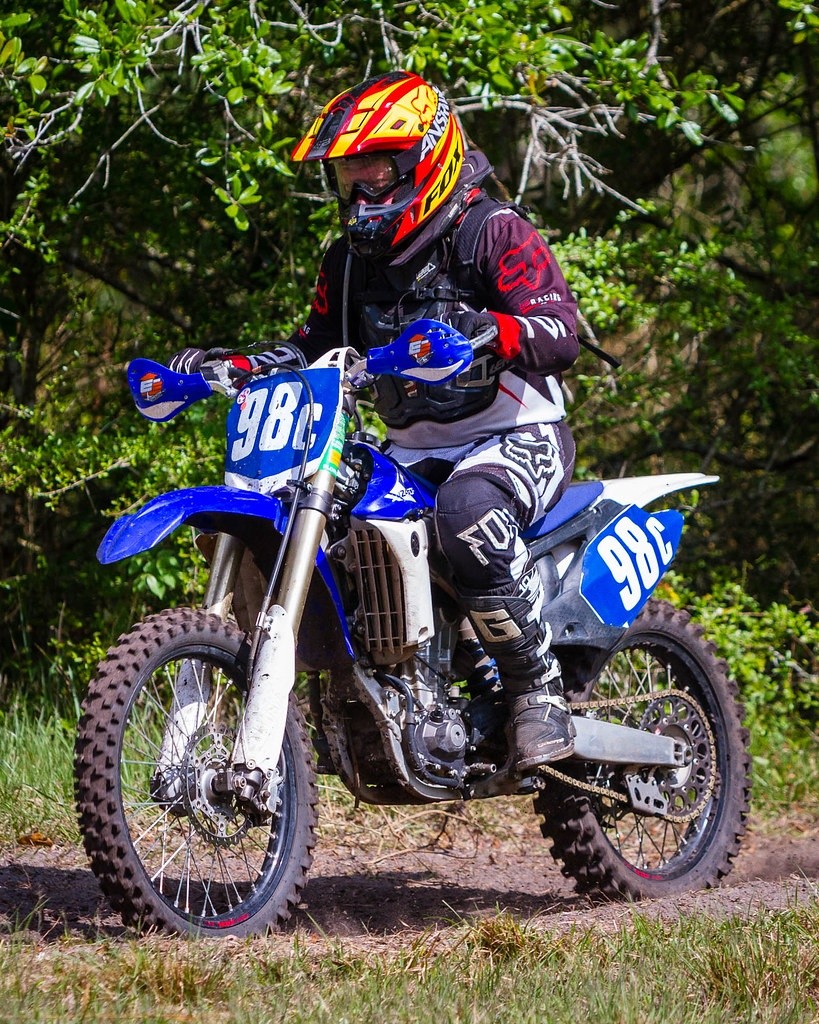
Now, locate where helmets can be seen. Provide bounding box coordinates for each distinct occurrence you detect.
[290,72,463,255]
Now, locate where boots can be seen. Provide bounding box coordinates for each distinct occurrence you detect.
[453,550,576,769]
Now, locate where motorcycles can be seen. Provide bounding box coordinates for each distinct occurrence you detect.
[70,319,754,939]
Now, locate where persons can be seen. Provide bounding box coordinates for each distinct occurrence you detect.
[169,72,576,772]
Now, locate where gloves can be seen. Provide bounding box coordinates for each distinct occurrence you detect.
[165,346,203,373]
[435,314,496,342]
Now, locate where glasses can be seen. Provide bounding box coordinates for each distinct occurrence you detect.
[328,153,400,208]
